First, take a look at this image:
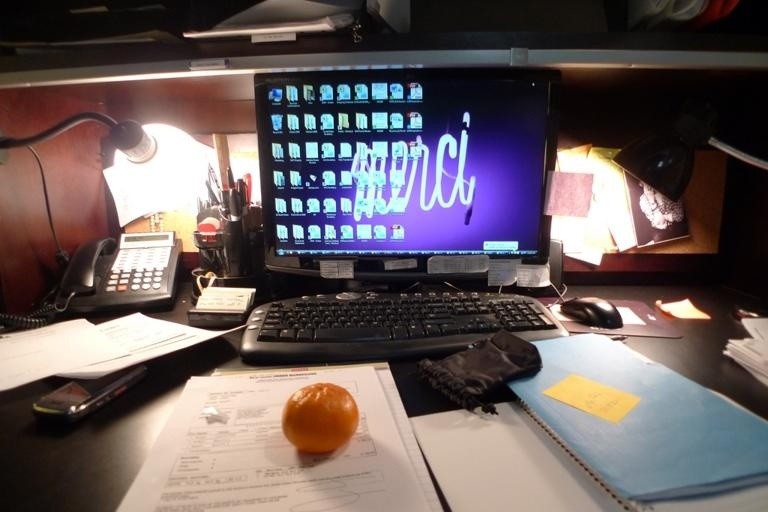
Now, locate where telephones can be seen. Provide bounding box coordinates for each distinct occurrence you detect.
[54,231,183,317]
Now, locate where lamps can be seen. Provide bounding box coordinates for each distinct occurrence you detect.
[610,99,768,203]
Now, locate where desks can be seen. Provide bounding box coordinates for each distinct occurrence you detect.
[2,274,766,512]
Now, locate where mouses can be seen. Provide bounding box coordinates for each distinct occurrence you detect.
[561,296,624,329]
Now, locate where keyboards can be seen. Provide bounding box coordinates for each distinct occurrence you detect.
[239,289,570,366]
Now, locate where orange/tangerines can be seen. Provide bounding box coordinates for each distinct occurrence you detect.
[281,382,359,454]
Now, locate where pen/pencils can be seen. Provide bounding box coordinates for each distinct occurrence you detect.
[197,162,252,222]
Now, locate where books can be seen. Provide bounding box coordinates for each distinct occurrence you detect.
[718,311,768,385]
[114,362,449,512]
[549,116,700,268]
[507,329,768,502]
[401,385,767,512]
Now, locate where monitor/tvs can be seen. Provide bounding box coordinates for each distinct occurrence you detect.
[253,64,562,293]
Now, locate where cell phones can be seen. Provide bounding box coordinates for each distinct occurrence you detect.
[32,365,149,420]
